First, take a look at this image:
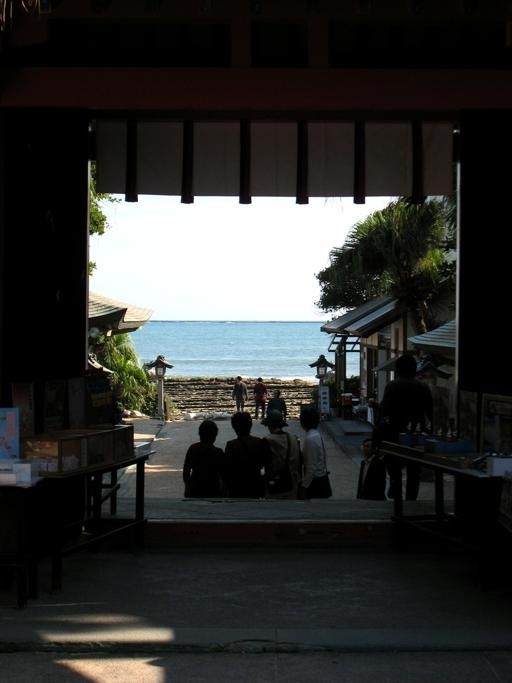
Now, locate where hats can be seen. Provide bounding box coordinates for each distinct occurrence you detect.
[261,410,289,427]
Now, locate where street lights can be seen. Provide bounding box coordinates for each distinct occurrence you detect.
[146,354,174,418]
[309,353,334,388]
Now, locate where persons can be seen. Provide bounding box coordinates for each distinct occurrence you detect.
[232,376,248,412]
[183,420,227,497]
[265,389,288,418]
[261,409,302,500]
[356,317,455,503]
[298,405,328,500]
[224,412,265,497]
[253,378,268,420]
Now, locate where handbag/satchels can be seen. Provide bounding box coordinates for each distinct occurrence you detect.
[266,465,294,494]
[306,476,332,499]
[297,482,307,500]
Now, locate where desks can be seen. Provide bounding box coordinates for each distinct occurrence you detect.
[14,446,158,593]
[377,440,511,572]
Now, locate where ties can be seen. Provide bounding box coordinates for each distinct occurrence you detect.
[363,462,369,484]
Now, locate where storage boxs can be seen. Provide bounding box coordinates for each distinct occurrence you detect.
[21,423,137,472]
